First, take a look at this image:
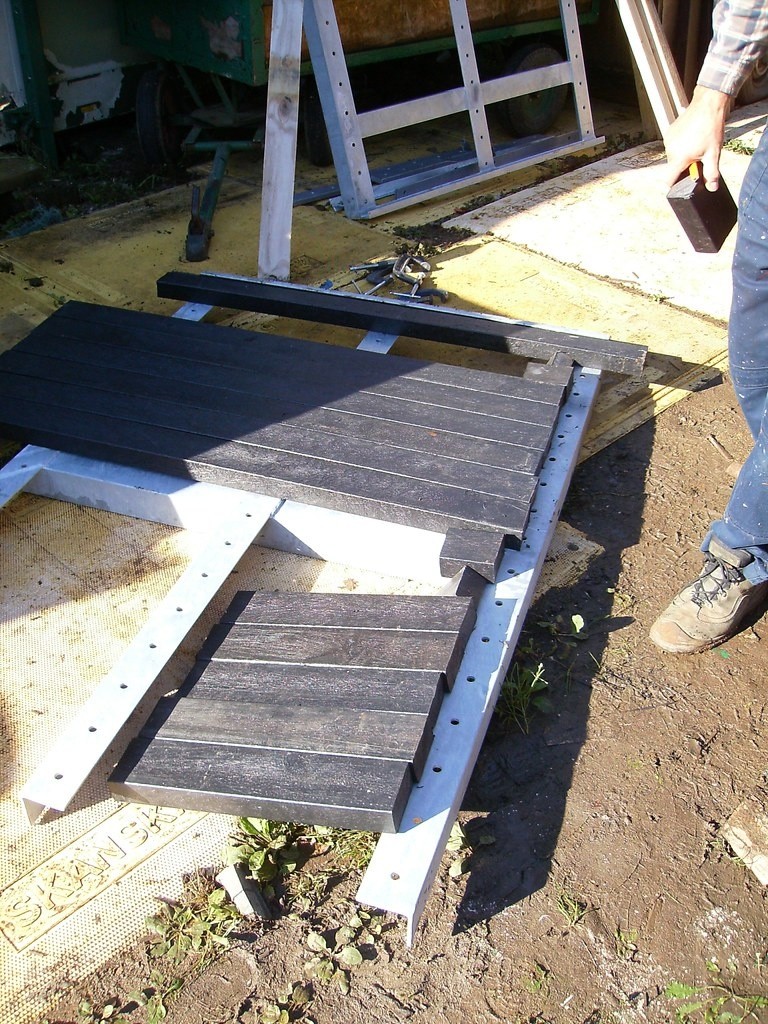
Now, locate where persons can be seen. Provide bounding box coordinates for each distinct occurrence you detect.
[647,0,768,654]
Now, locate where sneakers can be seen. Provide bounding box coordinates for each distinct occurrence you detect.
[648,537,768,653]
